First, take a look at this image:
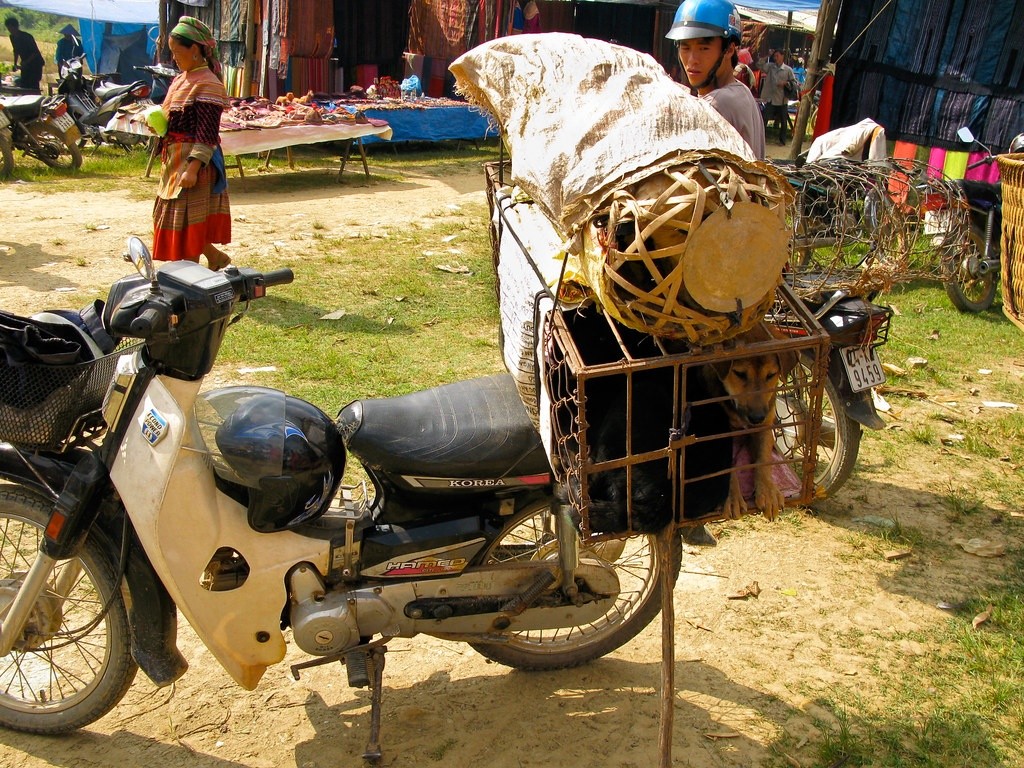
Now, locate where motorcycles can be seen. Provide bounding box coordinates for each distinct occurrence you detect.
[0,89,83,175]
[914,127,1024,312]
[748,259,893,505]
[0,238,685,765]
[766,118,886,275]
[52,34,152,158]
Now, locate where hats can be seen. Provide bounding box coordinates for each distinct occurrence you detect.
[59,23,79,36]
[314,83,384,100]
[295,102,369,127]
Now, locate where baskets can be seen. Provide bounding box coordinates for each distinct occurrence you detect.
[0,337,147,452]
[994,153,1024,332]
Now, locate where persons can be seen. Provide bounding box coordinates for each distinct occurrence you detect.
[56,23,83,79]
[4,17,45,96]
[145,16,232,271]
[665,0,765,162]
[733,48,806,146]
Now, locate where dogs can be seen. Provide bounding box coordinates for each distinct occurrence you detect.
[543,162,801,531]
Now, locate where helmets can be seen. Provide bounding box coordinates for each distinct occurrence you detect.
[1008,133,1024,154]
[665,0,742,47]
[215,393,345,533]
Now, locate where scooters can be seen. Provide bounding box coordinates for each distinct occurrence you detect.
[103,64,179,156]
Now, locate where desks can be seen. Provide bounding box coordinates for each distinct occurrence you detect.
[310,100,499,157]
[104,106,393,185]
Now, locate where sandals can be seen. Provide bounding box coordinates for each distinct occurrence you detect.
[207,252,232,272]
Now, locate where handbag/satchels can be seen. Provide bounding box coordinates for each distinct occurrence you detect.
[784,79,801,101]
